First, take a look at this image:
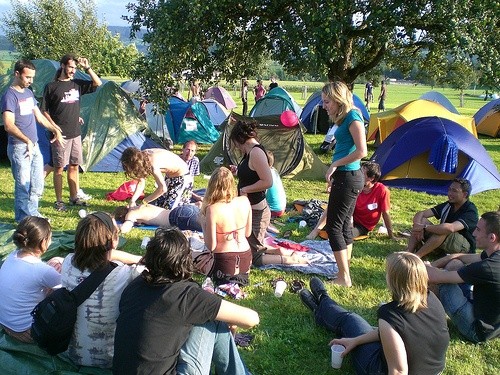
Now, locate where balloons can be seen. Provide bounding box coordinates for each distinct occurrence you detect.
[280,110,298,127]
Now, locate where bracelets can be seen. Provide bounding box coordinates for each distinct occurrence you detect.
[141,199,148,205]
[85,66,91,72]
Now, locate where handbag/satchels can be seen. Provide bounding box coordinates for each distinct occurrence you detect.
[28,259,117,355]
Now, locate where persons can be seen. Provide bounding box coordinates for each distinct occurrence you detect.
[322,81,368,287]
[406,178,479,259]
[43,68,84,179]
[153,140,201,189]
[62,211,150,367]
[112,226,260,375]
[253,78,277,102]
[241,81,250,116]
[39,55,102,211]
[423,212,500,344]
[227,119,312,266]
[364,78,374,112]
[0,216,64,344]
[118,147,204,210]
[264,150,286,216]
[300,251,450,375]
[114,206,204,234]
[0,59,68,223]
[190,168,253,278]
[377,82,386,113]
[304,161,402,240]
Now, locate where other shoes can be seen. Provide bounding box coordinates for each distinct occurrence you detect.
[309,277,329,303]
[68,197,86,206]
[54,201,69,213]
[298,289,318,314]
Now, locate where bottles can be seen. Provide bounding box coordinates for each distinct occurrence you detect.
[202,277,214,294]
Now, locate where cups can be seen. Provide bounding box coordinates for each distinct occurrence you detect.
[140,236,150,249]
[274,281,287,297]
[331,345,345,369]
[78,209,86,218]
[300,221,306,228]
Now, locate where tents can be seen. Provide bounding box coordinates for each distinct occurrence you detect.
[197,87,500,196]
[0,58,237,174]
